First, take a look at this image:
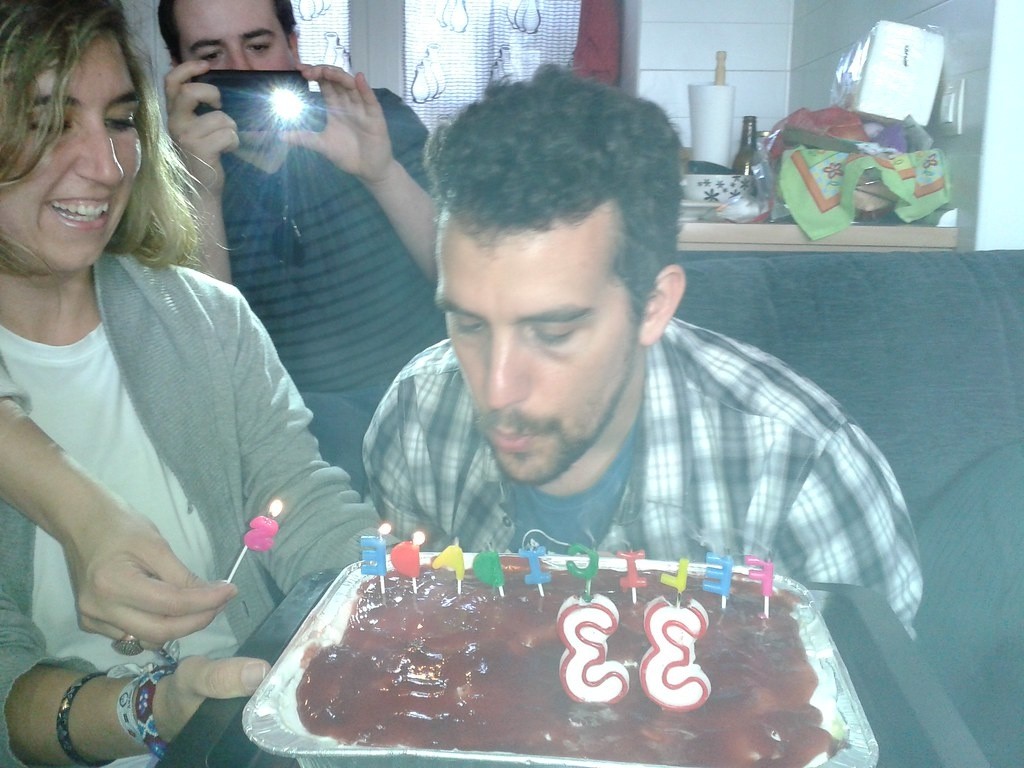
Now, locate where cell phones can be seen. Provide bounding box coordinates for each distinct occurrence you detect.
[190,68,327,132]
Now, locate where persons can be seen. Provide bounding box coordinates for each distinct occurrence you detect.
[0,352,241,653]
[154,1,452,499]
[0,0,378,767]
[359,65,923,640]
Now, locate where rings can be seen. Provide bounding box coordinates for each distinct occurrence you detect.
[111,629,142,652]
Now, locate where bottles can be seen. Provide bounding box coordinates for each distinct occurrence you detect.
[733,116,761,176]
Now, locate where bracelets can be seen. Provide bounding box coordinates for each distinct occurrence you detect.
[117,665,173,763]
[57,671,117,767]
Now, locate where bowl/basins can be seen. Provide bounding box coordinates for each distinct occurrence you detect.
[682,175,750,203]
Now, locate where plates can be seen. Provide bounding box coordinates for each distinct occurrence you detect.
[677,203,718,222]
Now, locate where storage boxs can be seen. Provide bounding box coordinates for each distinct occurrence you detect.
[829,20,944,127]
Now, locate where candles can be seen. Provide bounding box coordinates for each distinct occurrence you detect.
[360,523,775,596]
[244,500,283,551]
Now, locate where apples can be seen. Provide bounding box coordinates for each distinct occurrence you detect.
[825,123,869,142]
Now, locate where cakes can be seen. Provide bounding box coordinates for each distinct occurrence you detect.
[271,554,851,768]
